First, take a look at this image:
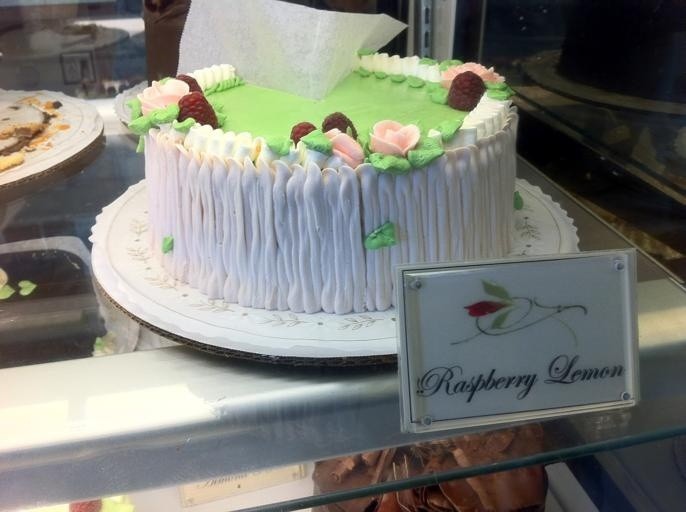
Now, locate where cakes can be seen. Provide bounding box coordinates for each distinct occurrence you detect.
[126,44,520,316]
[311,422,548,512]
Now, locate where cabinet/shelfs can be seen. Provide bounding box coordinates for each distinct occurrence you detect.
[0,0,686,512]
